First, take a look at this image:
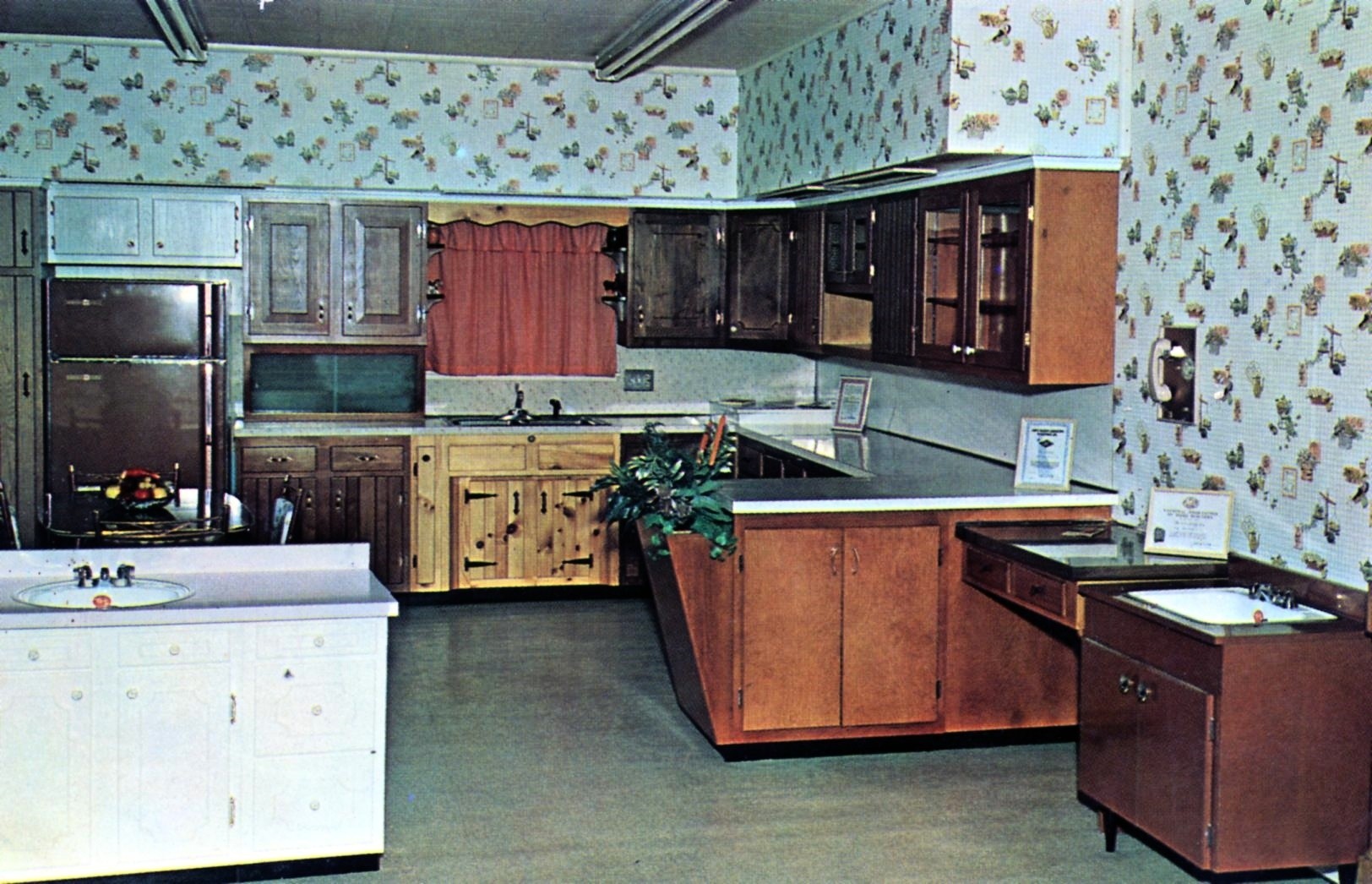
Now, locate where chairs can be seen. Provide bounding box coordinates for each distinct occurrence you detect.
[262,473,308,547]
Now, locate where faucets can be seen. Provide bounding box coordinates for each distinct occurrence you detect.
[1271,588,1299,610]
[509,391,529,416]
[97,568,114,586]
[1249,583,1276,603]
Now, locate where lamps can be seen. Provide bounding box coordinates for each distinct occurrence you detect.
[584,0,742,86]
[132,0,213,69]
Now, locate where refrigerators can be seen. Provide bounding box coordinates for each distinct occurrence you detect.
[45,278,227,511]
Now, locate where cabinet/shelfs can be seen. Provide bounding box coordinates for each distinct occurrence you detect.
[0,159,1372,884]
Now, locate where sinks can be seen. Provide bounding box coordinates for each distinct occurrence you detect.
[11,576,197,610]
[446,414,612,427]
[1121,587,1339,626]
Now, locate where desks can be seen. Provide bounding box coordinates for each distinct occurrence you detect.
[33,485,257,542]
